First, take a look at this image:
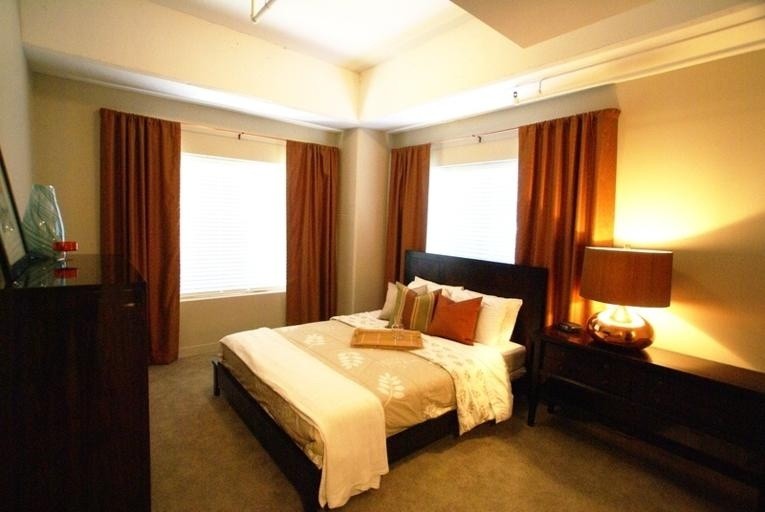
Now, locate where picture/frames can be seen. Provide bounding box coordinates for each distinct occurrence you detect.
[0,148,32,285]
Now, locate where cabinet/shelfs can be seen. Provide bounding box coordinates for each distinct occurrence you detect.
[0,252,152,511]
[526,322,764,491]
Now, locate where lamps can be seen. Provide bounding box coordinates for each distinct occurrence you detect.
[579,246,674,351]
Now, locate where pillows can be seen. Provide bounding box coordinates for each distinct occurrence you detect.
[376,276,523,350]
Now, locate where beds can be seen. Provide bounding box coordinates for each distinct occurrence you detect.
[210,249,549,512]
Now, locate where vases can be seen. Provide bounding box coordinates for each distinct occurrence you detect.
[21,183,64,260]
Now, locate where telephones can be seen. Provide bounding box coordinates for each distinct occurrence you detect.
[555,319,583,335]
